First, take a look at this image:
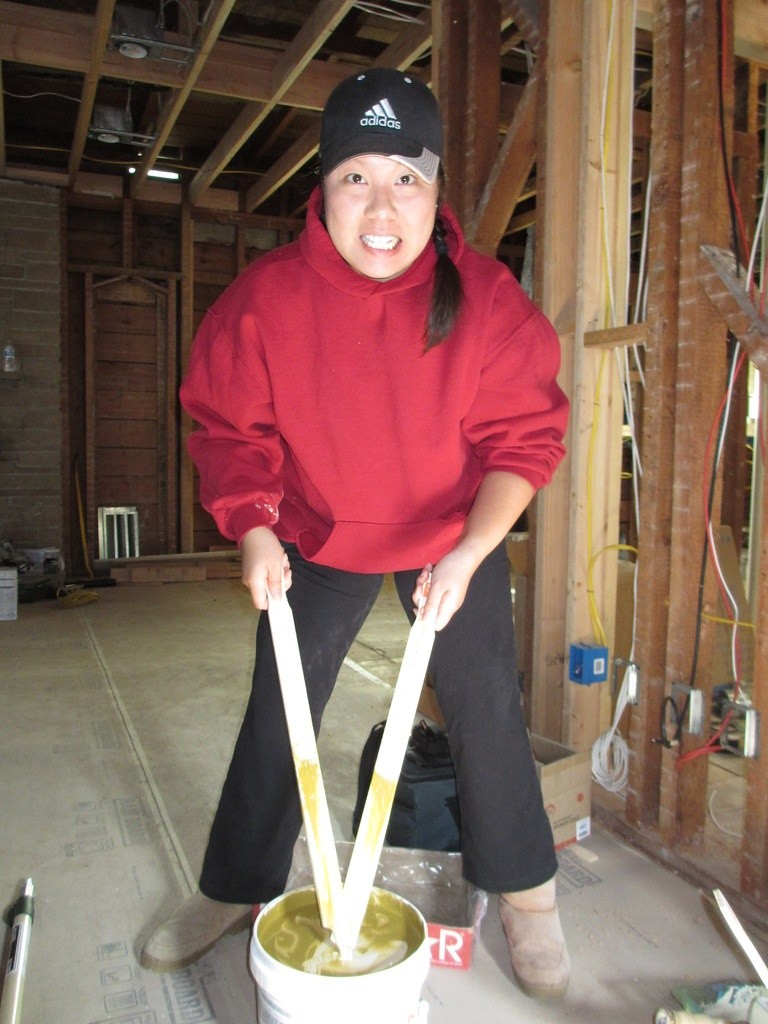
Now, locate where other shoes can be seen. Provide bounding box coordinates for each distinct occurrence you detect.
[140,882,266,974]
[499,882,568,1001]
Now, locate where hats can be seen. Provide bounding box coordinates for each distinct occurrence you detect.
[319,66,447,188]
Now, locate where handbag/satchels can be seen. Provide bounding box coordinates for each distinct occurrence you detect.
[352,721,461,852]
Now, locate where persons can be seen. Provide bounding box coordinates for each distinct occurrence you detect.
[139,68,576,1002]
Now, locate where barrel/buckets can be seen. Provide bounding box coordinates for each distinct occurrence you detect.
[248,884,431,1024]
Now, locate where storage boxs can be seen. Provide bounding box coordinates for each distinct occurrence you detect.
[420,532,635,746]
[252,837,487,970]
[0,566,18,621]
[17,548,65,573]
[528,726,592,851]
[705,751,746,858]
